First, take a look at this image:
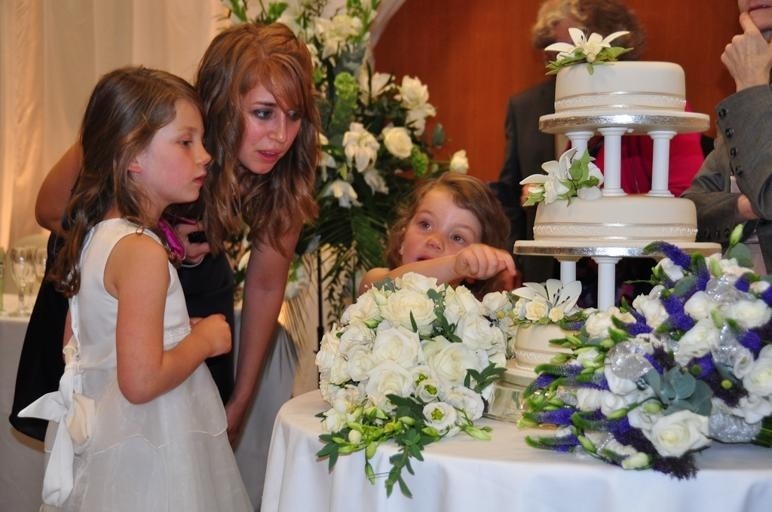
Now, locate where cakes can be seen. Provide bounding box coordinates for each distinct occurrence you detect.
[544,27,687,114]
[519,147,699,242]
[511,278,578,371]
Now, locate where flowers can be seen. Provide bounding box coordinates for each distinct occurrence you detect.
[212,0,469,303]
[512,219,772,482]
[481,291,520,360]
[314,271,518,500]
[544,27,635,77]
[519,146,602,209]
[512,278,587,327]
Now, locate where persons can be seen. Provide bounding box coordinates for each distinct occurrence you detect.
[358,169,518,297]
[9,24,321,512]
[19,65,254,510]
[489,0,772,282]
